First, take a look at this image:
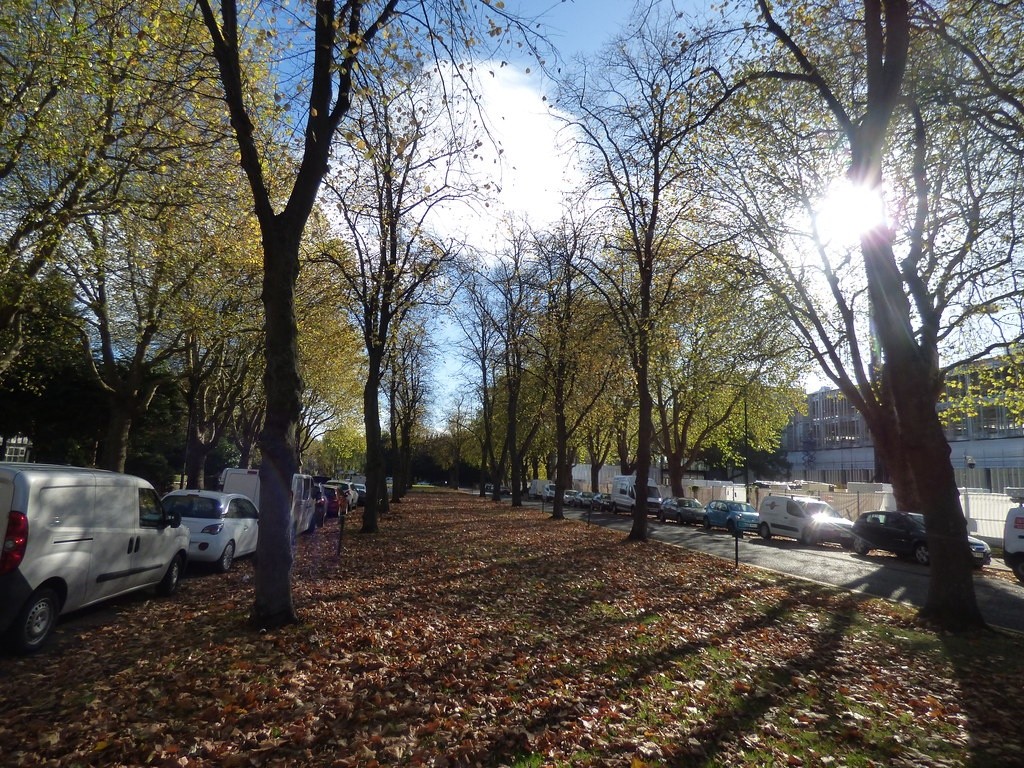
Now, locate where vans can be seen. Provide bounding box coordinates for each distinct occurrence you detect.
[758,492,856,549]
[611,475,663,520]
[0,461,191,654]
[529,480,553,500]
[221,469,317,545]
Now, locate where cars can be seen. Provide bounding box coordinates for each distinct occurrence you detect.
[852,511,990,571]
[659,496,707,526]
[161,489,260,572]
[703,500,761,536]
[563,490,612,512]
[1003,508,1024,583]
[543,485,555,500]
[315,480,366,527]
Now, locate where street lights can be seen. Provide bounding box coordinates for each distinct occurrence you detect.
[707,378,749,502]
[490,359,557,396]
[448,421,484,453]
